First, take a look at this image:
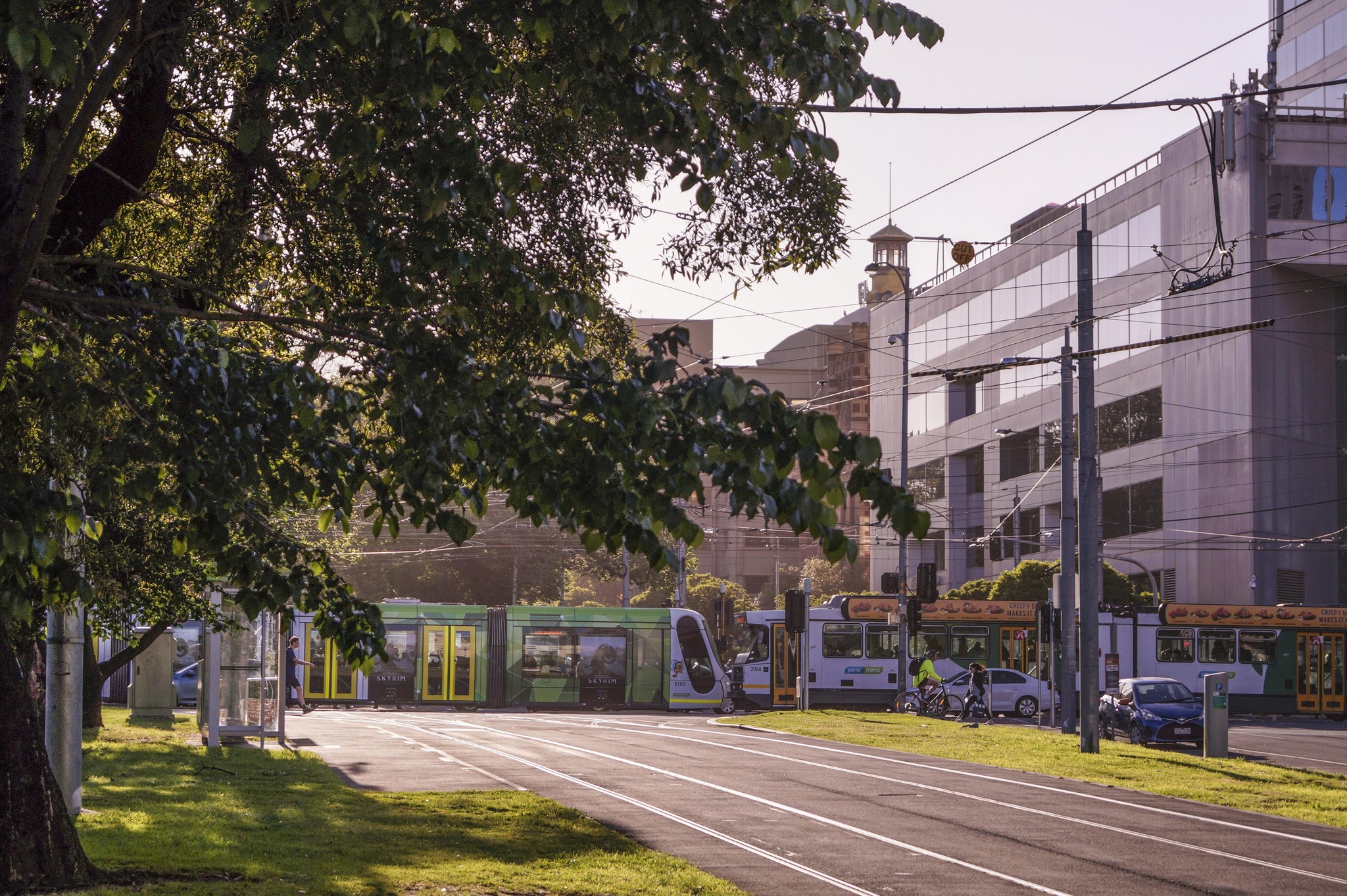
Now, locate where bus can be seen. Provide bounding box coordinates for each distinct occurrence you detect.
[730,595,1347,725]
[280,588,736,718]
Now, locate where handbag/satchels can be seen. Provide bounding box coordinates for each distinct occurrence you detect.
[972,699,985,718]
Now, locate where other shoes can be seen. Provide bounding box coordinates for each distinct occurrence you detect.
[956,718,965,723]
[333,706,340,709]
[397,706,405,709]
[983,720,994,725]
[346,706,354,709]
[922,712,933,716]
[920,696,931,705]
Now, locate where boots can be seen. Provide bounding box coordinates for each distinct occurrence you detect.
[298,704,314,714]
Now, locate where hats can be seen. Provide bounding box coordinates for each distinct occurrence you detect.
[957,637,966,643]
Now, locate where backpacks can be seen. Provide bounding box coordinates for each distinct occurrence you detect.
[909,657,934,676]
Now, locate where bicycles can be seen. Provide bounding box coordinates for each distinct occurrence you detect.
[894,680,964,721]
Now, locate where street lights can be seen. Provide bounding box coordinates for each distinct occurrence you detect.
[864,259,909,715]
[994,428,1078,734]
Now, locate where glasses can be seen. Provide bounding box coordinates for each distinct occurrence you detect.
[969,667,975,670]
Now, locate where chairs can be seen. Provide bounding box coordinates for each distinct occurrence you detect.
[1145,689,1156,701]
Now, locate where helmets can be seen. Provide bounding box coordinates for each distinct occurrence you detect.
[926,649,940,656]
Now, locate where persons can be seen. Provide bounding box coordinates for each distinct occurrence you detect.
[726,658,734,670]
[526,651,557,667]
[1040,651,1047,662]
[1309,652,1340,666]
[956,663,994,725]
[1001,641,1010,660]
[1160,648,1193,661]
[369,643,415,709]
[684,653,698,686]
[922,635,945,657]
[692,660,698,669]
[826,644,855,656]
[1027,639,1036,662]
[869,644,897,657]
[286,636,315,714]
[1230,646,1252,660]
[333,704,353,710]
[953,638,984,658]
[913,650,947,714]
[1211,640,1227,662]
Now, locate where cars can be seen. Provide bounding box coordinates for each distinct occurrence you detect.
[173,658,273,708]
[905,667,1061,718]
[1098,676,1204,749]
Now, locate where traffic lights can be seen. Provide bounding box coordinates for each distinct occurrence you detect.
[906,599,922,636]
